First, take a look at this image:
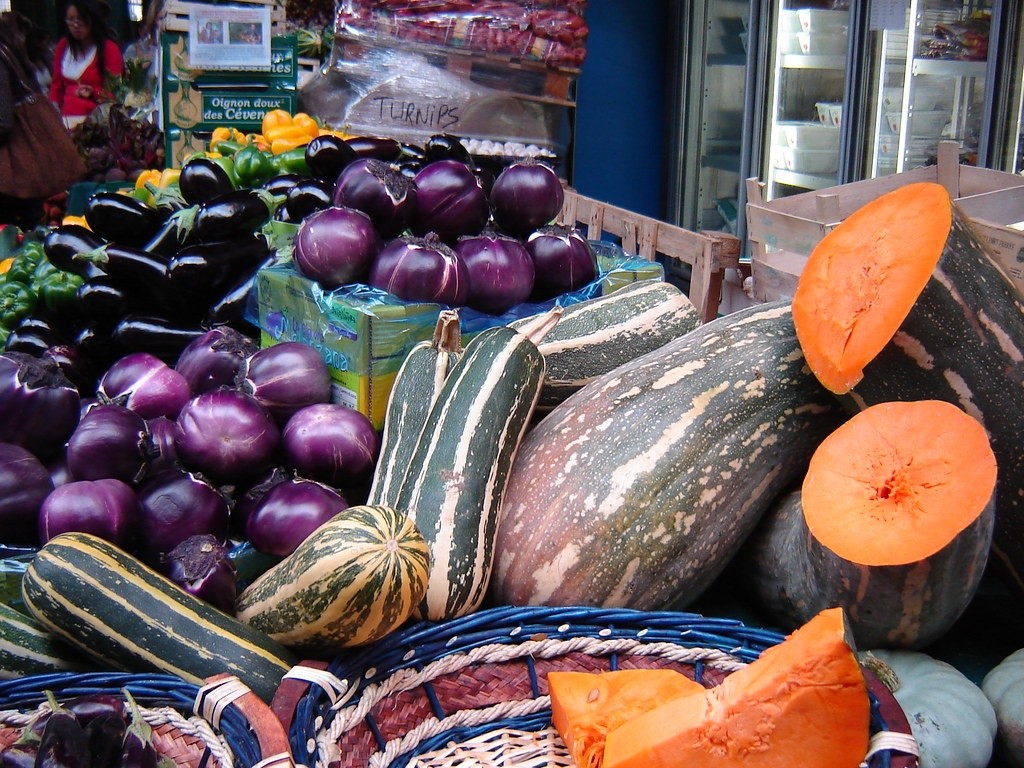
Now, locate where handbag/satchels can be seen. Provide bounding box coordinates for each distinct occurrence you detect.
[0,44,89,197]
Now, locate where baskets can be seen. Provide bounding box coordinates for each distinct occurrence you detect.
[293,605,919,768]
[0,672,294,768]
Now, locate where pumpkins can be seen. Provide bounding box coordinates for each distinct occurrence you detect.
[0,505,430,707]
[369,181,1024,768]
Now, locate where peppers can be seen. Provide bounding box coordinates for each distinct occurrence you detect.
[0,109,376,355]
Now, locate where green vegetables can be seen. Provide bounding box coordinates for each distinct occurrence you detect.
[294,27,323,56]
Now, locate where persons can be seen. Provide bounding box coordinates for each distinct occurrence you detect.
[200,22,222,44]
[0,0,162,229]
[237,24,261,43]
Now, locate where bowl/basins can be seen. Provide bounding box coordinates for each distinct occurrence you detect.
[880,86,953,167]
[773,102,842,171]
[779,8,849,54]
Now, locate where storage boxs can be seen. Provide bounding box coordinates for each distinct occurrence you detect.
[748,138,1023,306]
[255,256,665,431]
[158,0,298,168]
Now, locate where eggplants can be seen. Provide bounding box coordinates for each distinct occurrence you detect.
[0,157,380,618]
[0,686,165,768]
[258,134,599,316]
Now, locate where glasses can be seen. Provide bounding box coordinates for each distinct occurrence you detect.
[64,17,87,27]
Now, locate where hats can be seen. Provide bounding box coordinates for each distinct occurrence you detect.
[54,0,110,20]
